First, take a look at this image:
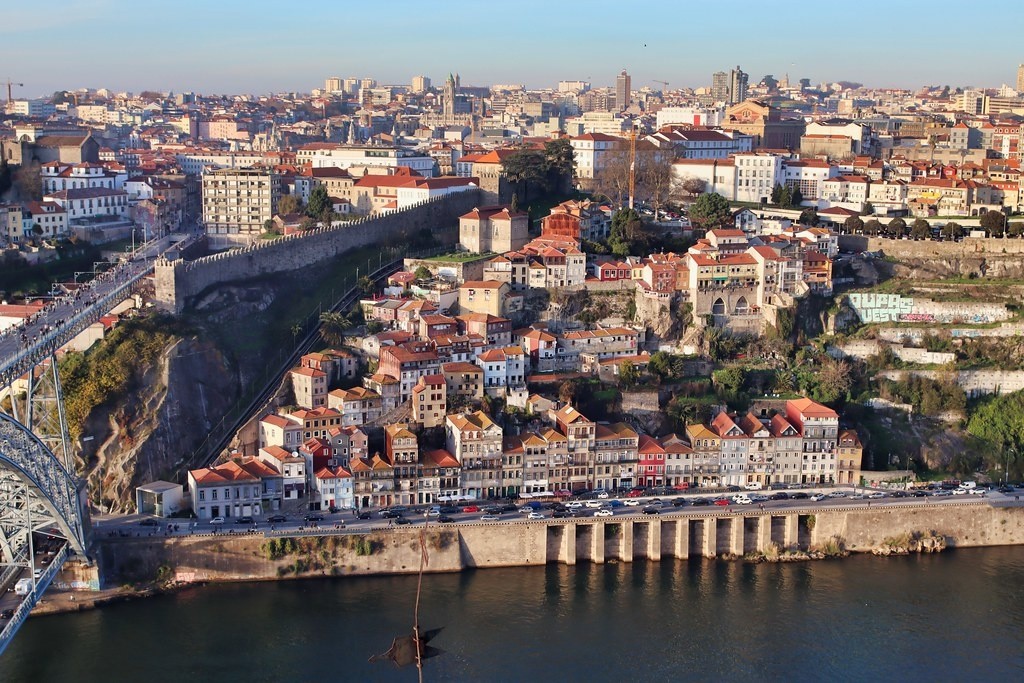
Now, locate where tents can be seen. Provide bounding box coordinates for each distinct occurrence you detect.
[438,495,472,501]
[519,491,573,498]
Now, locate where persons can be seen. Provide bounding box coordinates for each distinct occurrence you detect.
[12,263,131,348]
[724,493,930,512]
[112,506,393,533]
